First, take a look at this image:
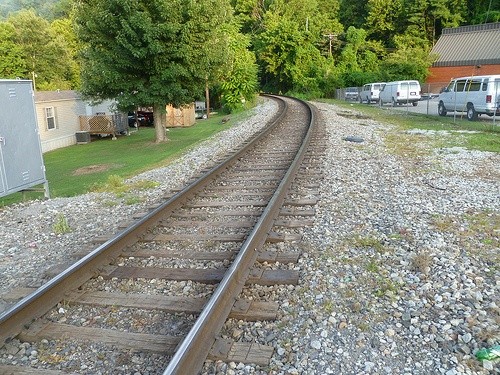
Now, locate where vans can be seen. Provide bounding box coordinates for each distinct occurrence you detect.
[438,75,500,120]
[359,83,386,104]
[345,87,359,101]
[378,80,422,109]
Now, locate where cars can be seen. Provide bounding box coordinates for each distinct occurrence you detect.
[134,109,153,126]
[194,109,208,119]
[128,111,150,128]
